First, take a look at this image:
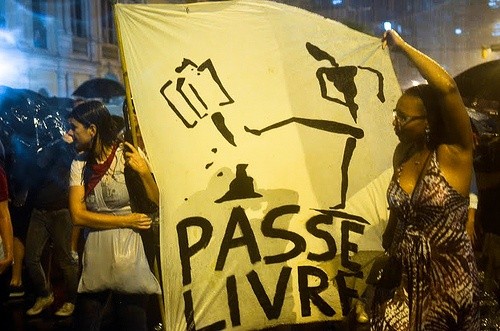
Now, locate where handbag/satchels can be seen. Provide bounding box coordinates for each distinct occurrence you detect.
[366,254,403,287]
[78,228,161,294]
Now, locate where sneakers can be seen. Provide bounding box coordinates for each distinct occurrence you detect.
[54,302,75,316]
[27,291,55,316]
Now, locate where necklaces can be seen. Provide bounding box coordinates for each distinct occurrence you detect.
[414,153,429,165]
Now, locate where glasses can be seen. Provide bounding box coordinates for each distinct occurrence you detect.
[392,109,429,126]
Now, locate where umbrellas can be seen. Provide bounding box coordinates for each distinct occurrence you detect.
[73,78,127,98]
[453,59,500,100]
[0,88,72,154]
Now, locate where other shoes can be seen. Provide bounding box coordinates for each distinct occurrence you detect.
[10,283,25,297]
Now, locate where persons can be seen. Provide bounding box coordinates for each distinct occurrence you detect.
[460,96,500,302]
[68,100,160,331]
[371,29,484,331]
[1,132,90,317]
